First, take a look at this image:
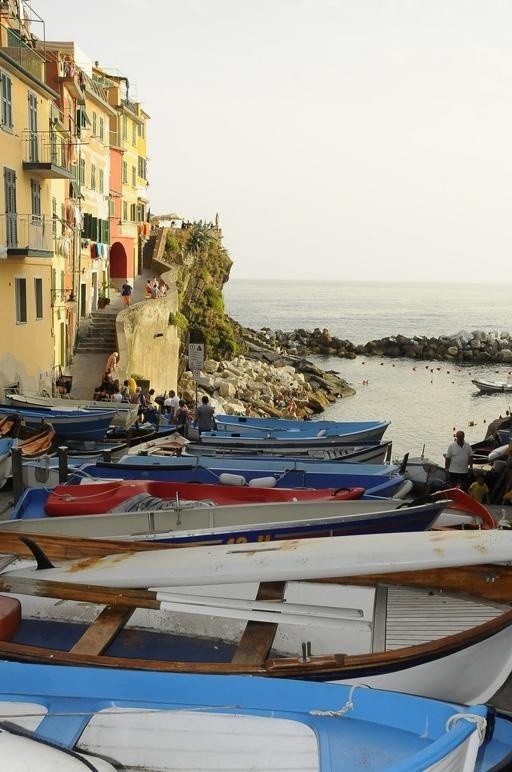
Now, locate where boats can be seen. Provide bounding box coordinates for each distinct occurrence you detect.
[1,530,512,706]
[2,394,512,510]
[0,659,495,772]
[4,487,498,536]
[470,378,511,392]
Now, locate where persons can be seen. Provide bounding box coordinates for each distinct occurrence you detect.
[170,219,193,229]
[445,431,473,493]
[468,473,491,505]
[94,351,215,436]
[121,279,132,309]
[144,275,168,301]
